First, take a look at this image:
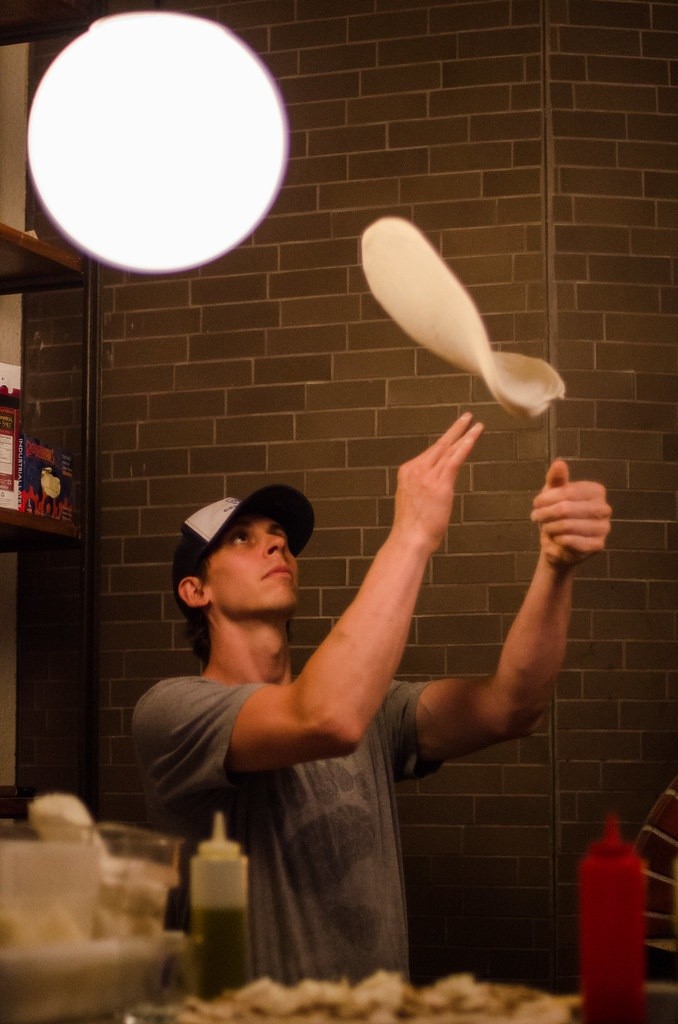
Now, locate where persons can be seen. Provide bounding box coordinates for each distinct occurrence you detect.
[130,412,610,1002]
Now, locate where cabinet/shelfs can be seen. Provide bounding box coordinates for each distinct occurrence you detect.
[1,221,101,821]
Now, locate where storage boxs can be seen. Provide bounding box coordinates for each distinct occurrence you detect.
[1,387,76,525]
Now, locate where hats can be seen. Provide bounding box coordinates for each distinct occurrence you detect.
[169,484,317,622]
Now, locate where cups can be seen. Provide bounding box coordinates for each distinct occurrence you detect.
[0,819,187,945]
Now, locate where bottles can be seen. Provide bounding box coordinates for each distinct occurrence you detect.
[189,812,252,1003]
[578,809,648,1024]
